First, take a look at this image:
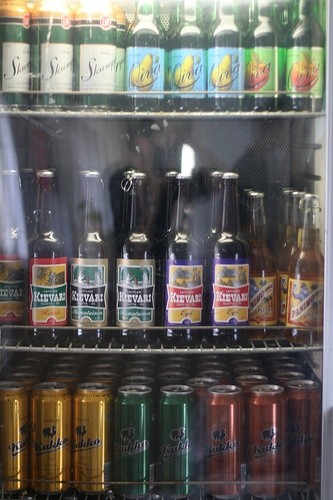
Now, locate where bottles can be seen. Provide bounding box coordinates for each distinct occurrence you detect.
[0,0,325,112]
[0,167,325,344]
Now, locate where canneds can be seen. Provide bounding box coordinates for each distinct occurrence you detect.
[0,356,322,499]
